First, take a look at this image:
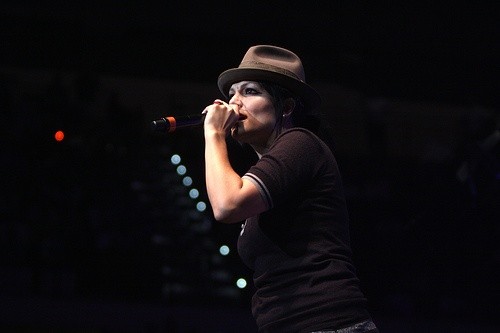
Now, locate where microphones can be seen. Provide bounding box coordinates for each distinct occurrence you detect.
[151,112,206,129]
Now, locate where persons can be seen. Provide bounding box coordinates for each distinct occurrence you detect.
[202,44,380,332]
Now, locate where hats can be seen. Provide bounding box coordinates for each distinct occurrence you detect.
[217,45,322,109]
